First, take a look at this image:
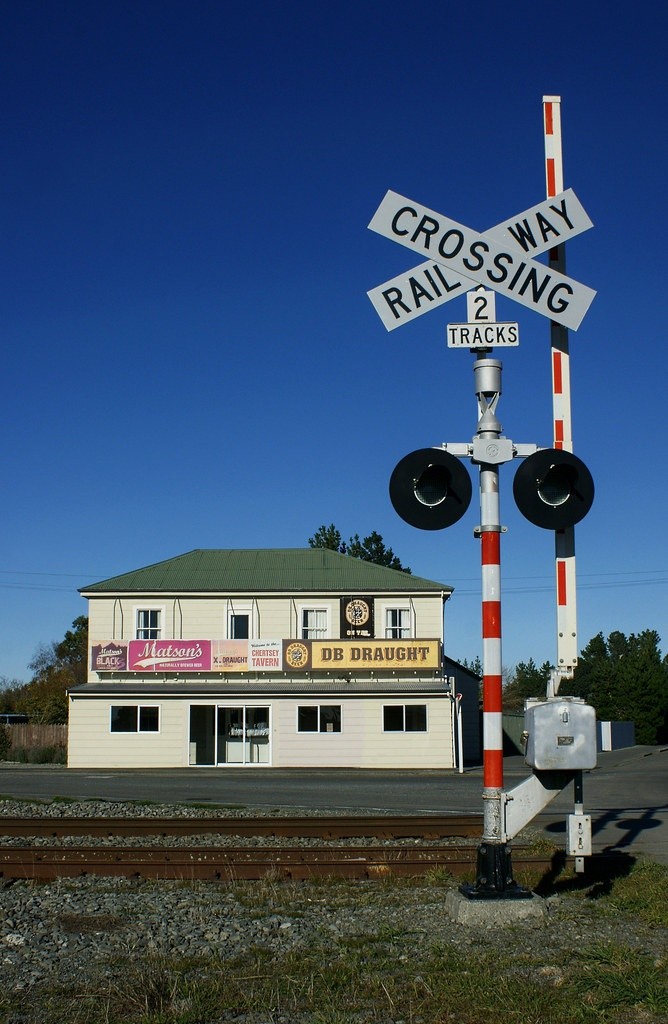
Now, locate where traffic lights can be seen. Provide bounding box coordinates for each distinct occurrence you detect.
[389,448,472,531]
[513,448,594,530]
